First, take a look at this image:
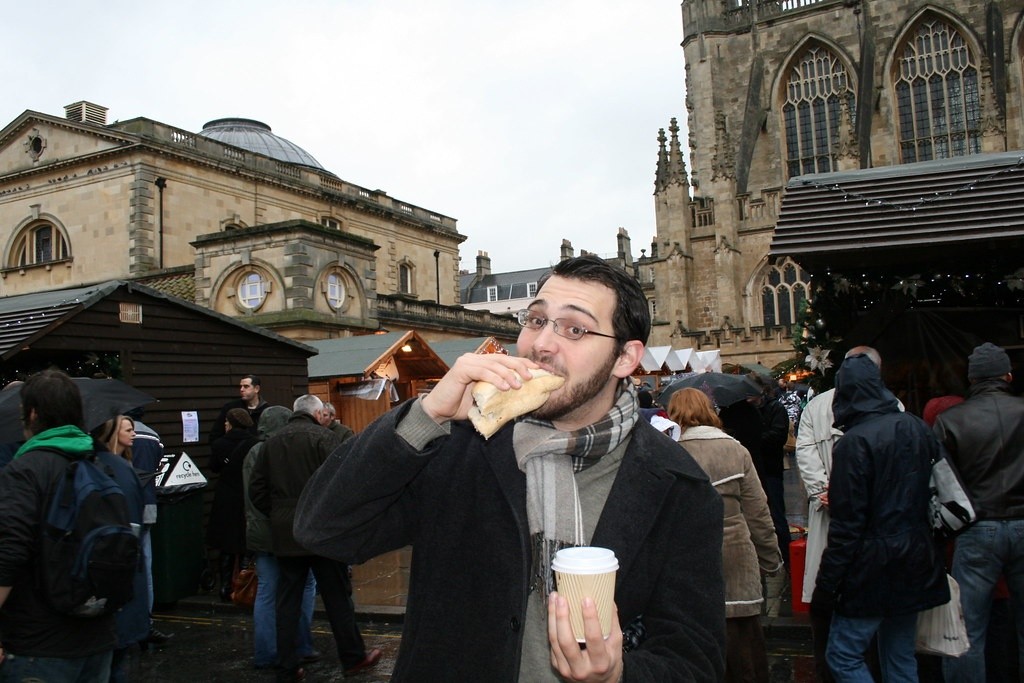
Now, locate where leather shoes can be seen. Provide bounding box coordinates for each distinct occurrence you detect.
[343,647,382,675]
[276,667,304,683]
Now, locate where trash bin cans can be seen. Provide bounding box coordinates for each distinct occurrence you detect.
[150,483,203,605]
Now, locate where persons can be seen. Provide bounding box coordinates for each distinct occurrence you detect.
[294,256,726,683]
[203,375,382,683]
[0,369,166,683]
[638,342,1024,683]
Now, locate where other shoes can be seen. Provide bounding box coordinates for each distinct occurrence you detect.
[297,651,320,661]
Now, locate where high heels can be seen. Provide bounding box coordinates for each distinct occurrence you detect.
[139,627,176,652]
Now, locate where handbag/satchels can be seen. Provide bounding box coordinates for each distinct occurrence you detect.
[930,456,975,536]
[918,573,973,659]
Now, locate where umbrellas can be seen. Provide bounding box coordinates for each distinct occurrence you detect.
[661,372,766,410]
[0,378,162,445]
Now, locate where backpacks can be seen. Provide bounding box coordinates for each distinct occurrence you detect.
[33,447,139,621]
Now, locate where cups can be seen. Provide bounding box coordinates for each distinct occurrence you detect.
[551,546,619,642]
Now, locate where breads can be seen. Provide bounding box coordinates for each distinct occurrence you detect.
[467,369,566,441]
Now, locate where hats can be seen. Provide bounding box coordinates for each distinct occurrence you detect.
[226,407,254,429]
[968,342,1012,378]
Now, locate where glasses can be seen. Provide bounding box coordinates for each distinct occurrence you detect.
[517,307,617,341]
